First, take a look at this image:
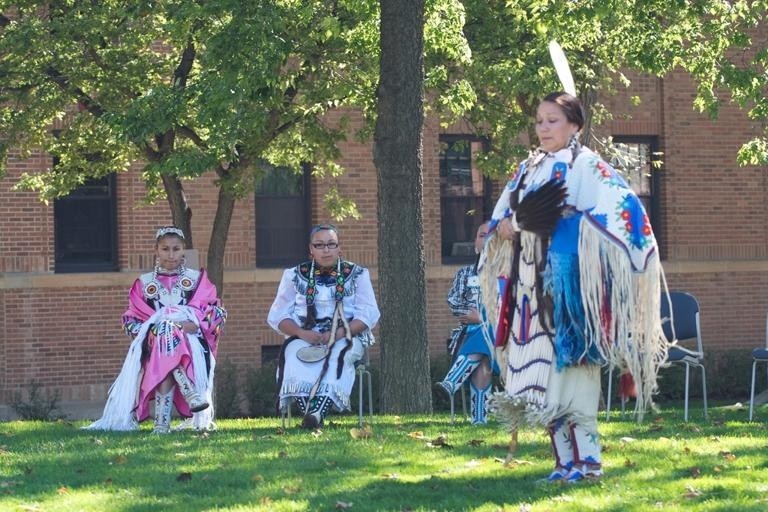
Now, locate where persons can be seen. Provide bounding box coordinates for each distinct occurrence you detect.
[433,220,505,427]
[264,221,381,431]
[472,90,673,491]
[119,226,228,435]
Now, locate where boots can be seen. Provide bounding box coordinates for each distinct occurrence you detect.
[535,413,604,484]
[434,353,483,404]
[153,382,177,434]
[172,365,210,412]
[469,381,493,426]
[293,396,333,429]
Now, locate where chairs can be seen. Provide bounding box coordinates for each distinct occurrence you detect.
[605,289,768,423]
[282,347,374,429]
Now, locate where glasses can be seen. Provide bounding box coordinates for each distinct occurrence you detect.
[311,242,339,249]
